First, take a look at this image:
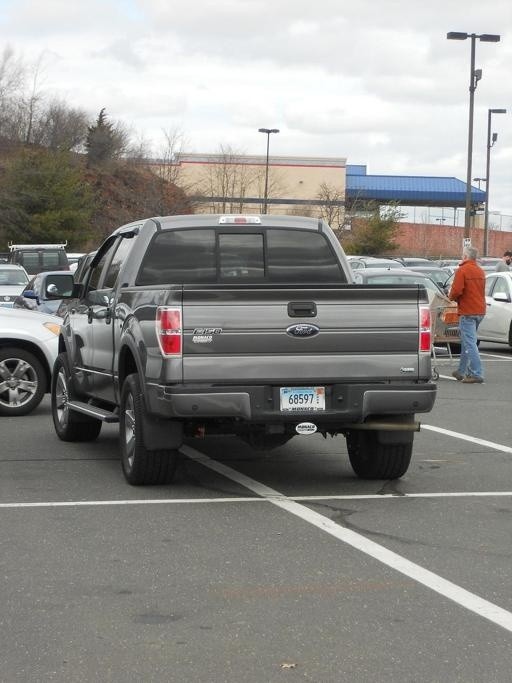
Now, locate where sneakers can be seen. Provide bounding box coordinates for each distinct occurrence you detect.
[451,369,466,380]
[461,375,484,383]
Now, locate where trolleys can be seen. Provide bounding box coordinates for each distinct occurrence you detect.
[431,306,461,381]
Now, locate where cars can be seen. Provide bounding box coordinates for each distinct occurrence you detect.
[0,240,97,416]
[345,255,512,354]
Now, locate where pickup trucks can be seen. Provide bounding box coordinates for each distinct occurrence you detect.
[45,214,430,486]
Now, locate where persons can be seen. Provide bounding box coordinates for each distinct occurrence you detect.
[448,244,486,382]
[502,251,512,262]
[506,254,512,264]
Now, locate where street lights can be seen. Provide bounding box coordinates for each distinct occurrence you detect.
[473,178,486,189]
[483,109,507,256]
[258,128,280,214]
[447,32,500,247]
